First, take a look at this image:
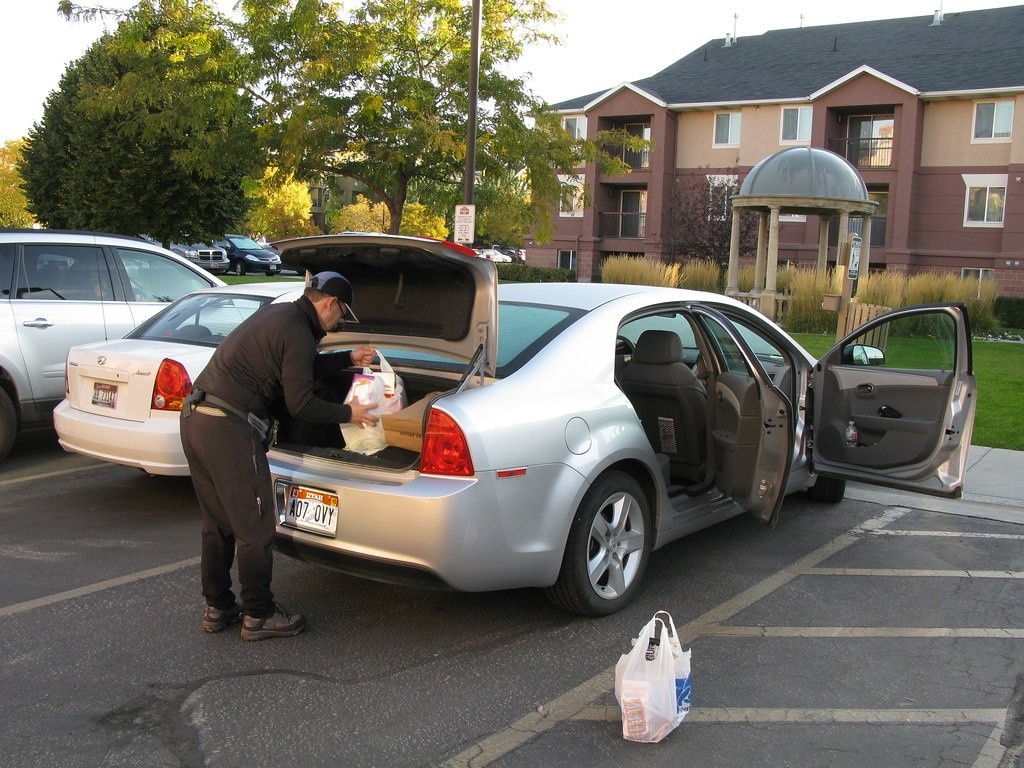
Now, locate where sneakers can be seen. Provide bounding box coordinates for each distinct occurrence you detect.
[202,602,242,633]
[239,602,306,642]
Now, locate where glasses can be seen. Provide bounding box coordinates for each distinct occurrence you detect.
[337,301,347,323]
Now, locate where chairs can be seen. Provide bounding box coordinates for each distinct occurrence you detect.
[618,330,711,483]
[37,263,72,294]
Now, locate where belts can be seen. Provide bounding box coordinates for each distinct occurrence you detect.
[190,404,228,417]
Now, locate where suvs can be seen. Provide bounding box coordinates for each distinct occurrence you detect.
[136,229,231,278]
[0,228,253,465]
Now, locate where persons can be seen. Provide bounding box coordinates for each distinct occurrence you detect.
[179,271,380,642]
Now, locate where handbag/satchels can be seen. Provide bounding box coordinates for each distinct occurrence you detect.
[614,610,692,743]
[339,348,408,457]
[247,412,280,453]
[380,391,442,453]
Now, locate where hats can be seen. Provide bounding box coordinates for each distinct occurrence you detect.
[307,272,361,323]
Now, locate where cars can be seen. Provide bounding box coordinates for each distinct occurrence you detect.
[50,280,310,493]
[470,249,527,264]
[212,233,283,278]
[238,228,978,617]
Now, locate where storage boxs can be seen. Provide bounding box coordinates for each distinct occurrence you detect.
[352,370,397,402]
